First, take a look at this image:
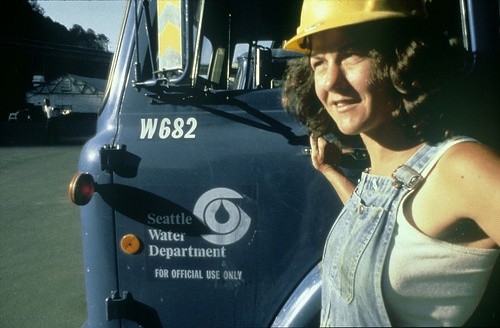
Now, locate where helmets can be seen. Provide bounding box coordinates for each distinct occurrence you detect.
[283,0,407,54]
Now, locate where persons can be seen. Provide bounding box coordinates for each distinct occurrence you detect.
[42,99,59,137]
[283,0,499,328]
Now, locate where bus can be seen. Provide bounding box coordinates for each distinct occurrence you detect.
[70,0,498,327]
